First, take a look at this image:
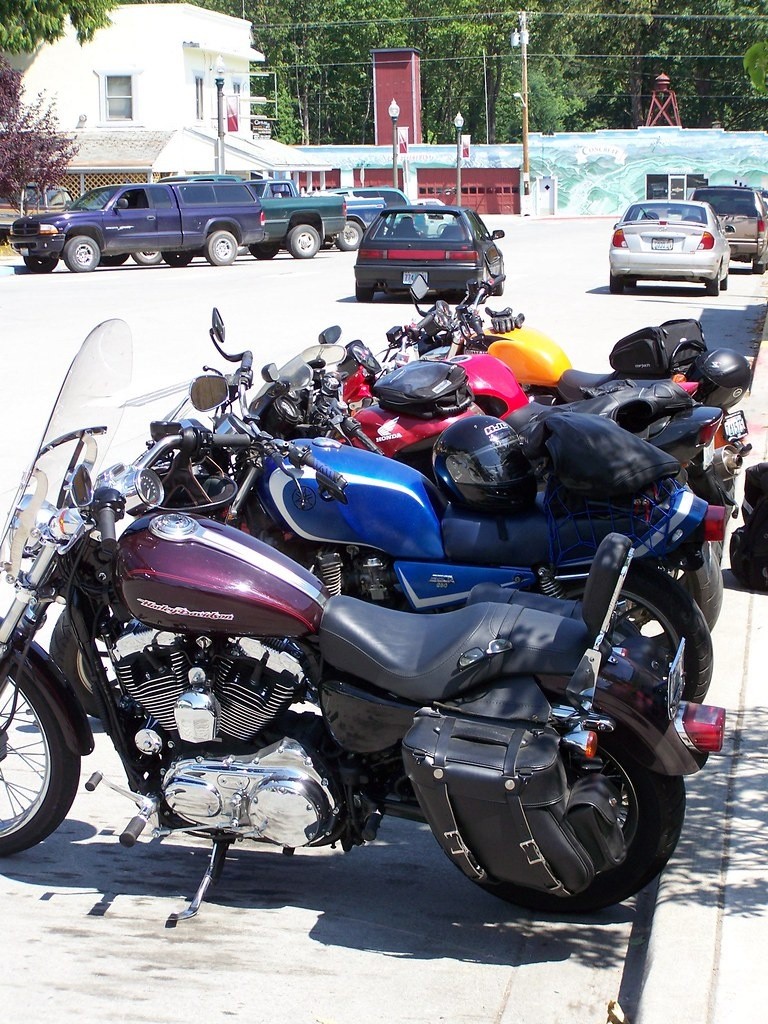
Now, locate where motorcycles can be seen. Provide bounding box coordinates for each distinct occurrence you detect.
[0,273,754,923]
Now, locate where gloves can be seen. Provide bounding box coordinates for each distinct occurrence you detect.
[485,307,525,333]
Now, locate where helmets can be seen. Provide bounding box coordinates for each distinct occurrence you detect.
[432,414,538,517]
[685,348,752,409]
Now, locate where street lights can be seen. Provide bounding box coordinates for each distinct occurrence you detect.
[388,99,400,191]
[510,9,531,196]
[213,56,227,176]
[454,112,465,207]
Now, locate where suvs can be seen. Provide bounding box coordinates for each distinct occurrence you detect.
[312,187,413,228]
[681,186,768,276]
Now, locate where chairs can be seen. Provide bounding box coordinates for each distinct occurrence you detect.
[440,225,464,240]
[641,213,660,220]
[684,214,701,221]
[395,222,420,239]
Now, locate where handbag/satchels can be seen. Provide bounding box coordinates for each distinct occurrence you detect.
[373,359,475,418]
[610,319,708,376]
[741,462,768,532]
[729,525,768,593]
[465,584,643,639]
[400,702,626,899]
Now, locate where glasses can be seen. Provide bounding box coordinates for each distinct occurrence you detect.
[456,639,512,672]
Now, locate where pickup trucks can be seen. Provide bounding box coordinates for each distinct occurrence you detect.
[7,180,269,274]
[243,178,387,252]
[100,175,347,266]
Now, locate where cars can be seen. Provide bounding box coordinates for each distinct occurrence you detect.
[353,205,505,305]
[609,199,736,298]
[12,181,76,207]
[411,196,456,237]
